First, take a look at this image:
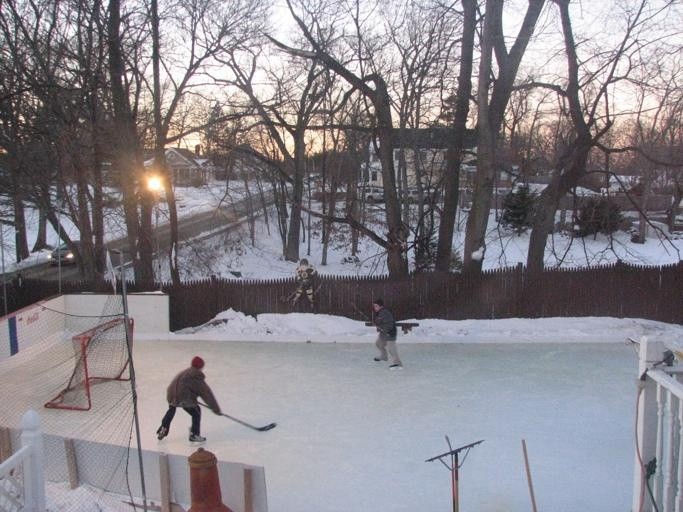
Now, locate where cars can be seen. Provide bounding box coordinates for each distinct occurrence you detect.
[459,188,471,192]
[361,187,386,203]
[49,239,82,266]
[401,190,429,204]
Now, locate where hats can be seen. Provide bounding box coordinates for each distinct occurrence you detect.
[373,299,383,307]
[192,357,204,368]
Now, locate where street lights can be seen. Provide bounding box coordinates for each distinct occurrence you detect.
[147,174,163,285]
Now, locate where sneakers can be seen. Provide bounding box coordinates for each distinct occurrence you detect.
[190,435,206,441]
[158,431,166,440]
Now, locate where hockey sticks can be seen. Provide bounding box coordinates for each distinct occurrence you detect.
[199,402,277,431]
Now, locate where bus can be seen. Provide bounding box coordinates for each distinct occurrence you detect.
[302,173,321,190]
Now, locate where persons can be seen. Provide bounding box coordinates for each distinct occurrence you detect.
[373,299,402,368]
[157,356,221,441]
[291,260,318,310]
